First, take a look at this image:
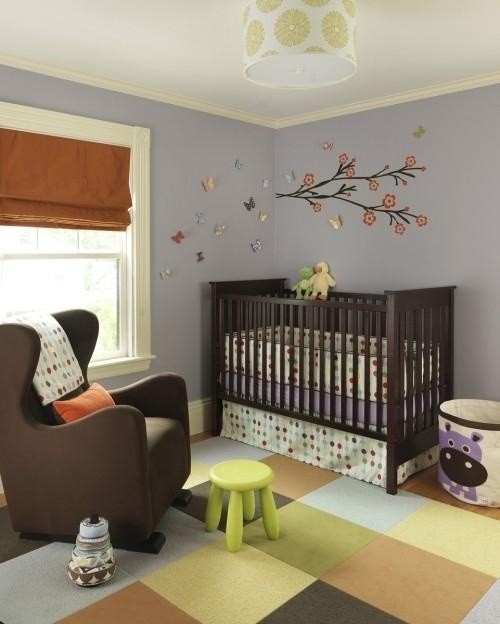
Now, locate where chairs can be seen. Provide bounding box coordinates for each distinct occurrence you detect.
[0,310,195,560]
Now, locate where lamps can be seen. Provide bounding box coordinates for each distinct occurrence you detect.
[242,1,358,91]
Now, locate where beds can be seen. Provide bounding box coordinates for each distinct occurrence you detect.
[209,277,456,495]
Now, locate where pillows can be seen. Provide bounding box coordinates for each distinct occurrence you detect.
[50,381,117,425]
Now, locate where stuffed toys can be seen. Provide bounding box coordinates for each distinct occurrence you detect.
[290,264,316,299]
[301,261,336,299]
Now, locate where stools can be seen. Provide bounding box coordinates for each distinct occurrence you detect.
[203,460,279,552]
